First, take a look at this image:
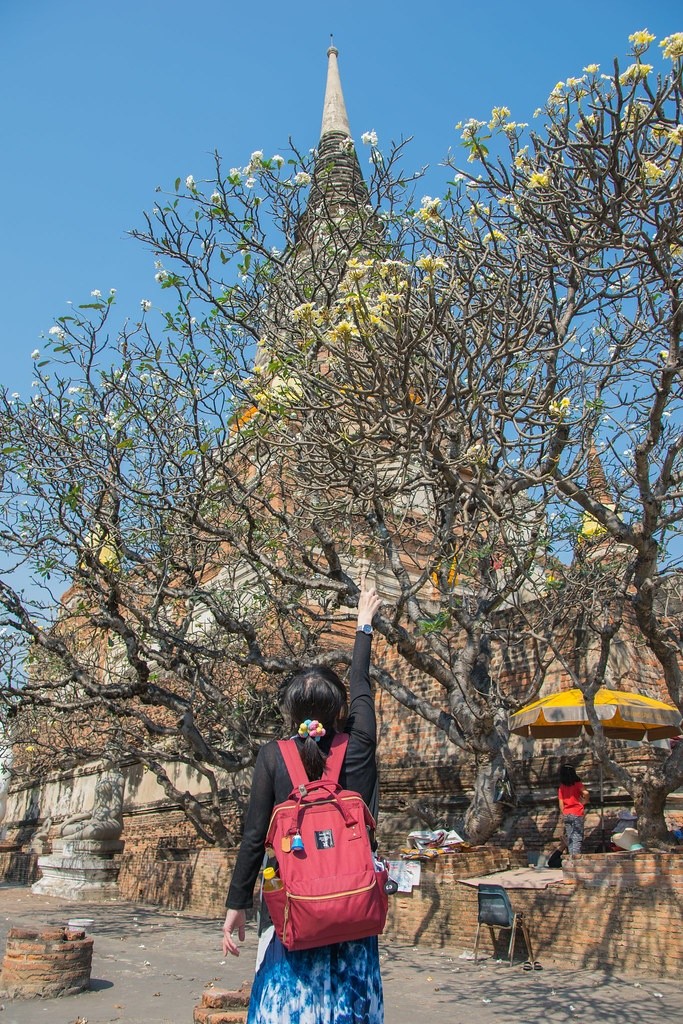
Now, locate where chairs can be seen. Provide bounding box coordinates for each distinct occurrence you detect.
[473,883,533,968]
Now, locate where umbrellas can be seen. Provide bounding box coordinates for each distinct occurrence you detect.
[507,689,683,853]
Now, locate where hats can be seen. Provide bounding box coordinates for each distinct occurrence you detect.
[611,809,644,853]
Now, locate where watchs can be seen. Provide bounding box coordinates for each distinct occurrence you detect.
[356,624,374,636]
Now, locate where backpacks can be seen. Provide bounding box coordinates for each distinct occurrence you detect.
[262,732,390,952]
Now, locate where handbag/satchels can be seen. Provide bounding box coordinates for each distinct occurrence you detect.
[492,769,518,808]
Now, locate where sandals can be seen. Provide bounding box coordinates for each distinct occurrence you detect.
[534,961,544,970]
[523,961,533,971]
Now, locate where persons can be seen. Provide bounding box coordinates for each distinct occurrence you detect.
[548,828,640,868]
[558,762,590,855]
[222,575,392,1024]
